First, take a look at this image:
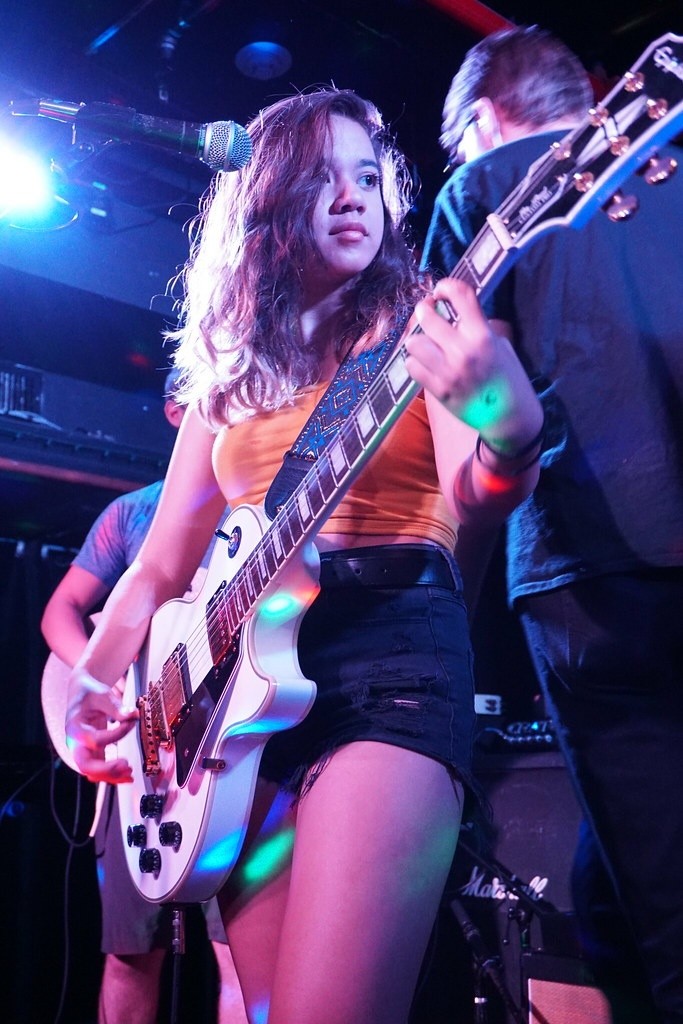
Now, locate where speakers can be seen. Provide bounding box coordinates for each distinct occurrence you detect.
[423,746,585,1023]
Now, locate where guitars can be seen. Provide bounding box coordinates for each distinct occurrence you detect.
[40,609,130,775]
[117,33,682,906]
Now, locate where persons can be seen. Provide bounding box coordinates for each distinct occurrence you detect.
[423,29,683,1023]
[41,347,253,1024]
[65,88,545,1021]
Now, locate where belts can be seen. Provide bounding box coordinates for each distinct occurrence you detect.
[322,553,455,589]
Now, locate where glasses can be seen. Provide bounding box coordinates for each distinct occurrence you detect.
[447,114,479,171]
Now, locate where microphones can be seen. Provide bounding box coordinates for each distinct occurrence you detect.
[9,95,254,172]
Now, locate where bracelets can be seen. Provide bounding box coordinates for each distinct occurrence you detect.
[476,425,545,478]
